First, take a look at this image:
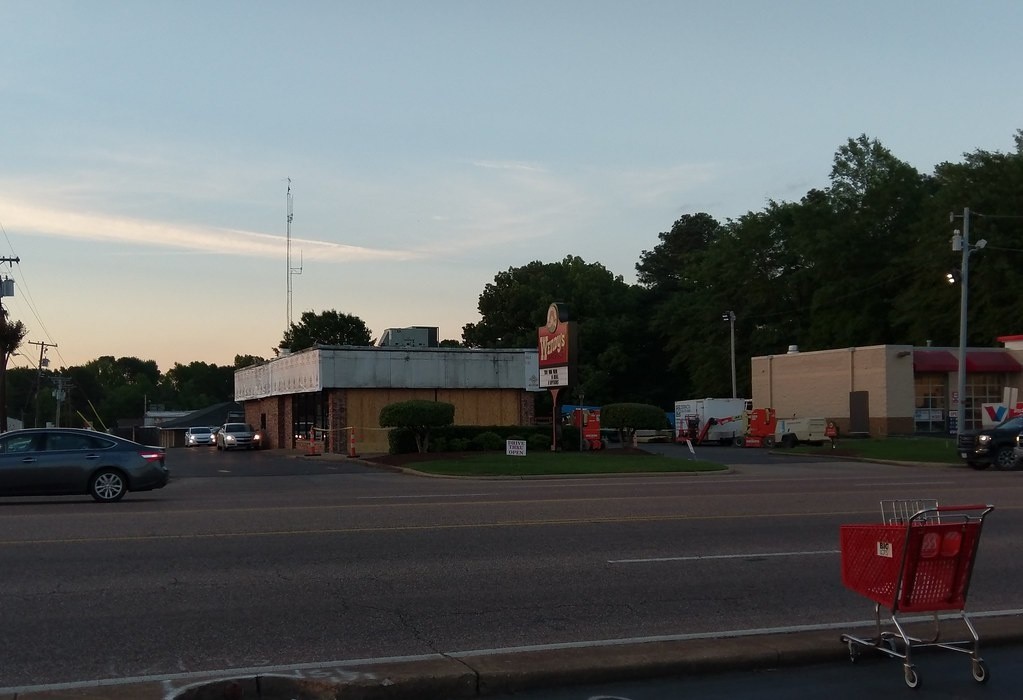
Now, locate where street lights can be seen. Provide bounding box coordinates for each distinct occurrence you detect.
[577,389,586,454]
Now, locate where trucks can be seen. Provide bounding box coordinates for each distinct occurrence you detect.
[674,398,752,447]
[735,407,831,450]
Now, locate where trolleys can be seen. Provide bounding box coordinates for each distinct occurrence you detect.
[840,498,990,690]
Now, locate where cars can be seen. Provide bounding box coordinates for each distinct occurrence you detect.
[216,423,262,452]
[0,427,170,503]
[185,426,216,448]
[601,427,674,446]
[957,415,1023,471]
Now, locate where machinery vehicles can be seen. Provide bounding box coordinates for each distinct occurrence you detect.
[675,413,746,448]
